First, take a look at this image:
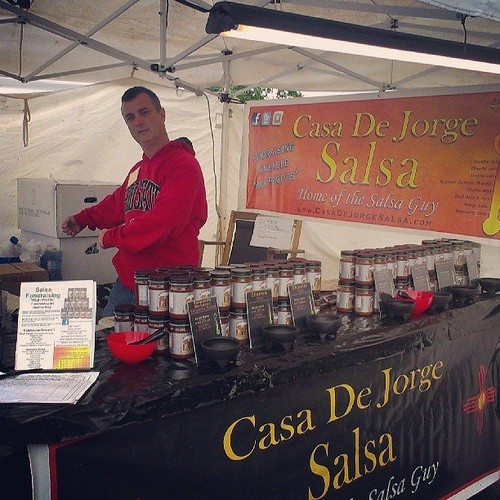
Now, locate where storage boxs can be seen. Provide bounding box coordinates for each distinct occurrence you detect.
[20,230,119,285]
[16,177,124,239]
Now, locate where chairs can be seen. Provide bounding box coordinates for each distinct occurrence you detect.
[198,210,306,269]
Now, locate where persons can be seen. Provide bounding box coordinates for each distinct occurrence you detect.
[62,85,208,314]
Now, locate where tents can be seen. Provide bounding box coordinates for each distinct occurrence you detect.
[0,0,500,280]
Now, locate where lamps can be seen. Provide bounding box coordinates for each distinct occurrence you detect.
[205,0,500,76]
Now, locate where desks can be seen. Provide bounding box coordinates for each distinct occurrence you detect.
[0,277,500,500]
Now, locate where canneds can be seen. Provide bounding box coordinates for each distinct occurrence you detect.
[113,257,321,359]
[334,237,482,315]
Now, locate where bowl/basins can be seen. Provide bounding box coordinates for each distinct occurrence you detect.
[480,277,500,297]
[200,337,241,366]
[396,290,434,316]
[384,299,415,321]
[430,292,453,312]
[261,324,300,355]
[106,331,157,364]
[305,314,342,338]
[451,285,478,306]
